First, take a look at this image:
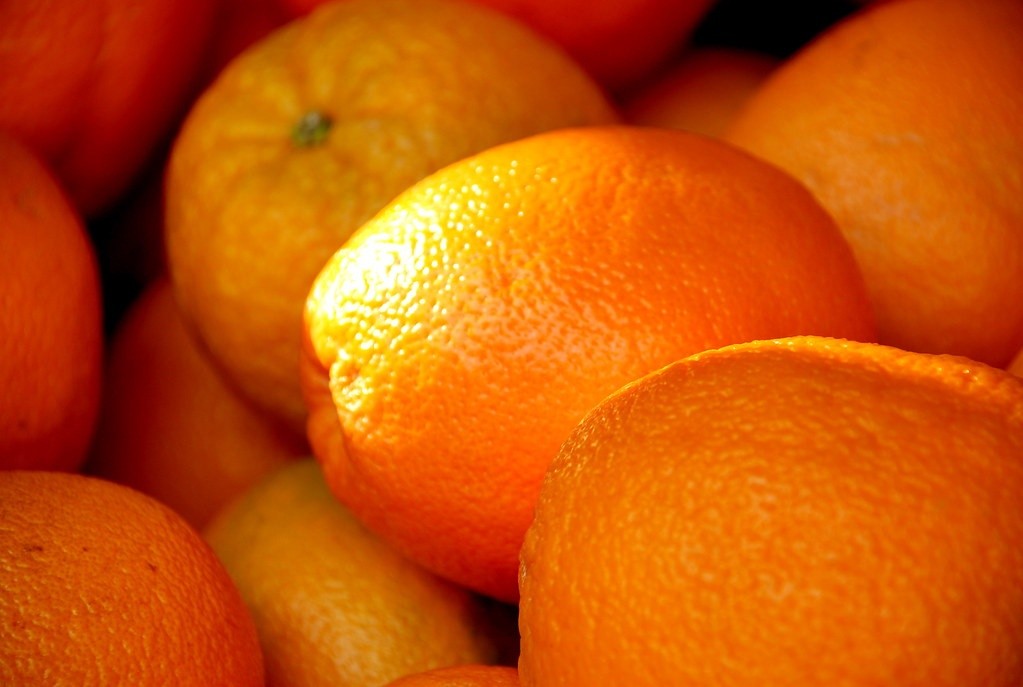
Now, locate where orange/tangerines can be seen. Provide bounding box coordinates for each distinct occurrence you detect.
[2,0,1022,687]
